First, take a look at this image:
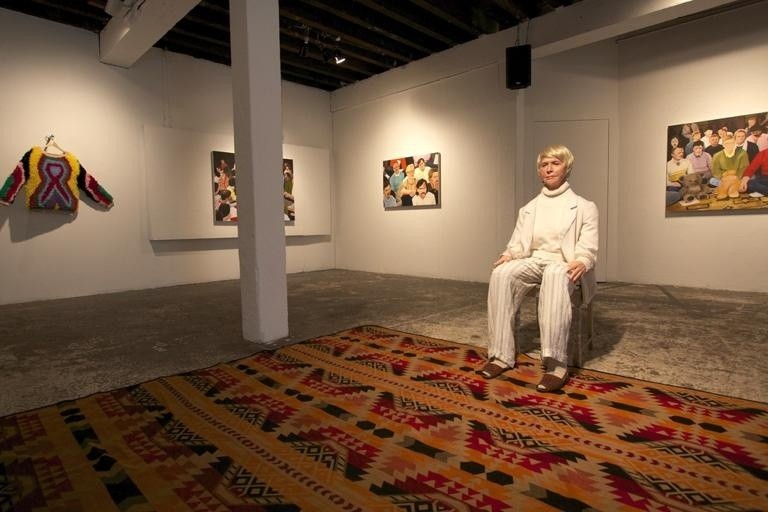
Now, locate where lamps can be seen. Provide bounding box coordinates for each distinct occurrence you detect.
[331,47,347,64]
[318,44,336,64]
[298,40,310,59]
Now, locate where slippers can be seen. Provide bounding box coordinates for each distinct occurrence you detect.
[480,361,510,378]
[536,368,569,391]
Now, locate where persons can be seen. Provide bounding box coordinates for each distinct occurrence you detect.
[666,117,767,207]
[213,159,236,222]
[479,146,599,393]
[382,158,439,208]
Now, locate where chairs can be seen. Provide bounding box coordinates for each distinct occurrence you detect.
[514,265,596,367]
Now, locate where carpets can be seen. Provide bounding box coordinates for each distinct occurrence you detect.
[0,324,768,512]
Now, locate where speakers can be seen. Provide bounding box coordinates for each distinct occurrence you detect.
[506,46,531,90]
